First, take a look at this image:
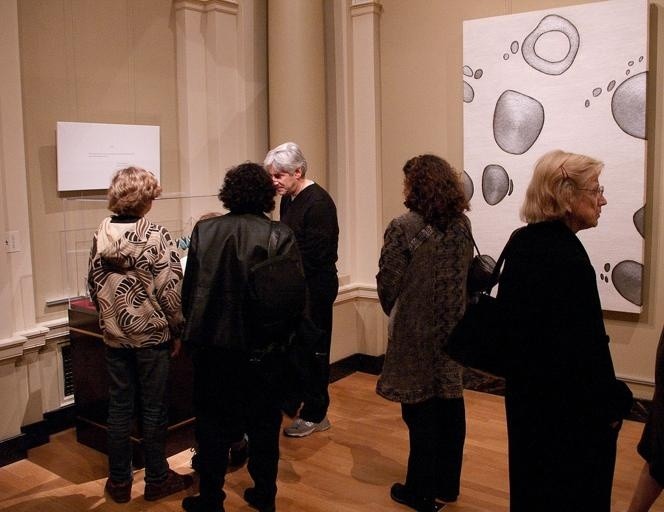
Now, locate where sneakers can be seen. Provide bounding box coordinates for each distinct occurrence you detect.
[284,416,330,437]
[106,476,133,504]
[143,469,193,502]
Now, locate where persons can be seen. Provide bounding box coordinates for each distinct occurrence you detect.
[87,167,193,503]
[376,153,475,512]
[496,150,626,511]
[628,324,664,512]
[263,142,340,438]
[191,212,250,475]
[181,163,308,511]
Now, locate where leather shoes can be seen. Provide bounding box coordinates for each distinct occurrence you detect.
[182,496,225,512]
[244,488,276,512]
[391,483,435,512]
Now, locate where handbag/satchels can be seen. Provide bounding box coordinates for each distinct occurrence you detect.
[248,254,307,364]
[444,294,503,380]
[466,255,499,294]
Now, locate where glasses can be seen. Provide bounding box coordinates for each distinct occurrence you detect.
[577,185,604,199]
